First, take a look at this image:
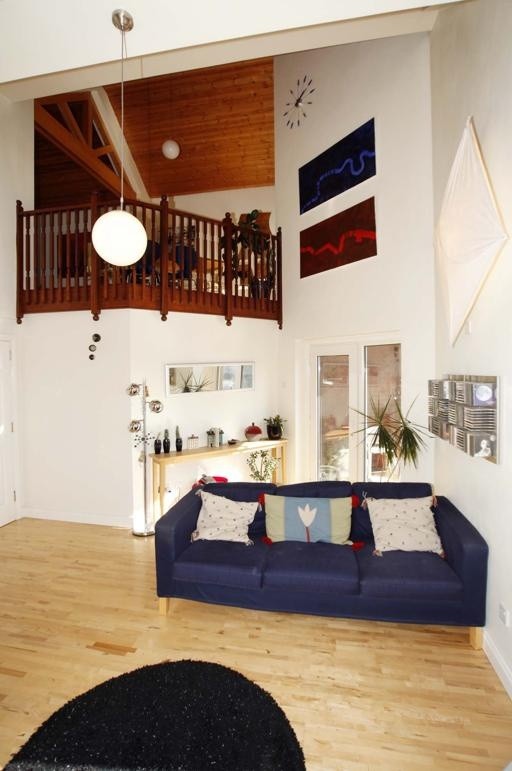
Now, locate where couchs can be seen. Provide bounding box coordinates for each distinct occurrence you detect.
[153,479,490,629]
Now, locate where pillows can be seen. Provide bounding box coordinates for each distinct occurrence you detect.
[362,495,448,557]
[190,488,261,546]
[258,491,365,550]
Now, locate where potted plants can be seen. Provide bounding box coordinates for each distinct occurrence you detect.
[263,414,288,441]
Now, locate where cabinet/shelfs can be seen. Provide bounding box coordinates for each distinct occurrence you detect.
[151,436,291,528]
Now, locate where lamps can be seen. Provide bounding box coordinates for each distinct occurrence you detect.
[89,8,147,267]
[161,138,181,160]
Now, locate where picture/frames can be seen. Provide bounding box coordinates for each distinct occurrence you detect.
[163,362,256,397]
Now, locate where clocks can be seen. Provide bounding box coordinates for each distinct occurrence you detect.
[281,73,317,129]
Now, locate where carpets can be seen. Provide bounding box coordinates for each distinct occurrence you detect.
[0,657,307,771]
